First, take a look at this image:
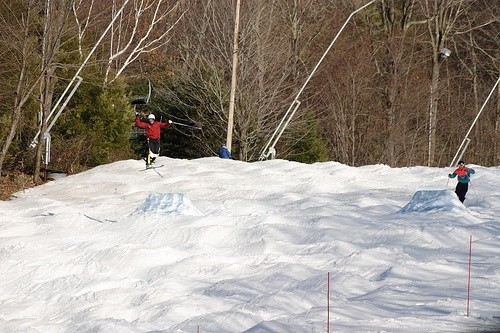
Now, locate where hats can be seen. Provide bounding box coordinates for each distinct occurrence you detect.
[147,113,155,119]
[458,160,464,164]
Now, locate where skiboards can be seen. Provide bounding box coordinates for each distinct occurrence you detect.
[139,157,164,178]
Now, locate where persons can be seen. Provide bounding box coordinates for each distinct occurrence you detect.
[218,143,231,160]
[135,112,173,170]
[448,160,475,204]
